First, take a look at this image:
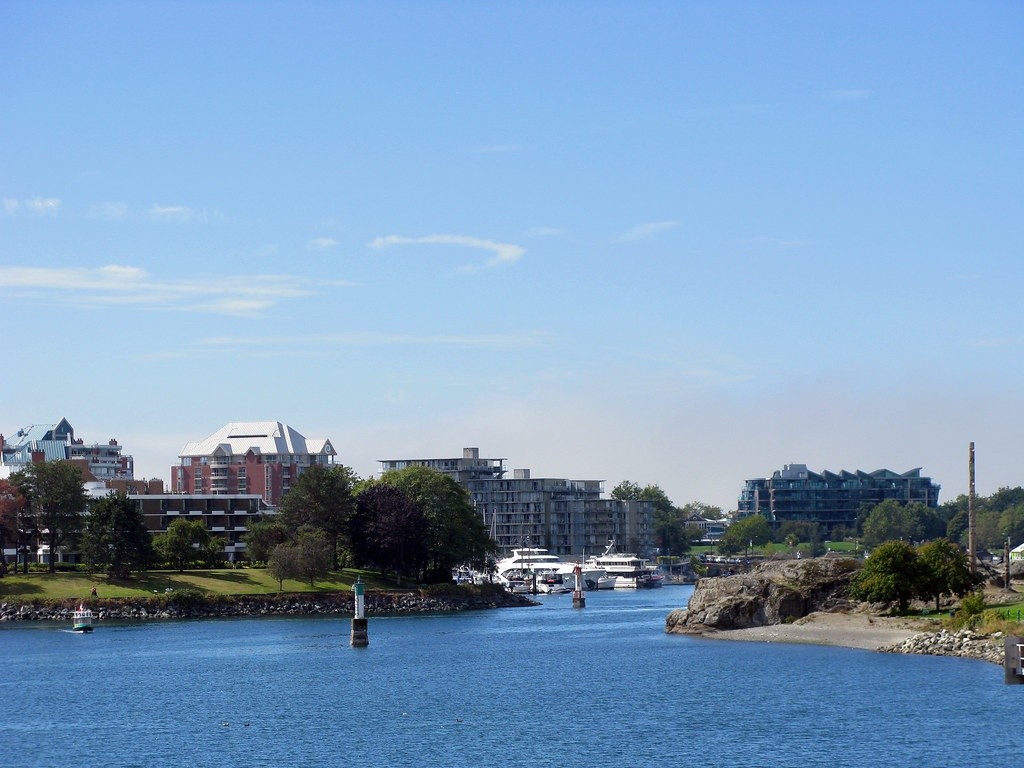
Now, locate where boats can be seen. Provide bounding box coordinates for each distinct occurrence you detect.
[452,499,665,590]
[71,606,94,633]
